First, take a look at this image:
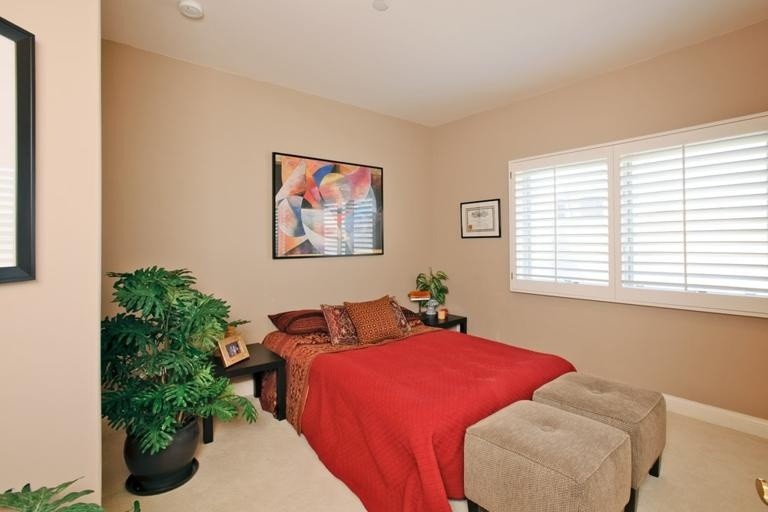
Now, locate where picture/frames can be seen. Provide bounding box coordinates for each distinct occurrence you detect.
[218,334,250,368]
[0,16,37,287]
[460,198,501,238]
[271,152,384,259]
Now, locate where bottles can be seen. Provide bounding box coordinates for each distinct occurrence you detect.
[440,307,448,318]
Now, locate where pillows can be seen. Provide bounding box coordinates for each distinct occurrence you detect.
[266,294,421,347]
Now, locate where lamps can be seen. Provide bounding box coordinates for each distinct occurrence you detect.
[408,291,431,321]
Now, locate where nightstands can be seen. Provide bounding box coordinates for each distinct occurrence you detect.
[413,311,467,332]
[202,343,286,445]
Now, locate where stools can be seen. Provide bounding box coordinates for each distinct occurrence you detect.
[463,399,632,511]
[532,370,668,512]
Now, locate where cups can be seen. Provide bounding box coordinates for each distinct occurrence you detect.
[438,310,445,319]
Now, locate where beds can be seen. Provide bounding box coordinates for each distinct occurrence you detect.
[263,318,577,512]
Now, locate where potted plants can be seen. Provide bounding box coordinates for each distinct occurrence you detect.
[414,267,447,315]
[101,265,258,496]
